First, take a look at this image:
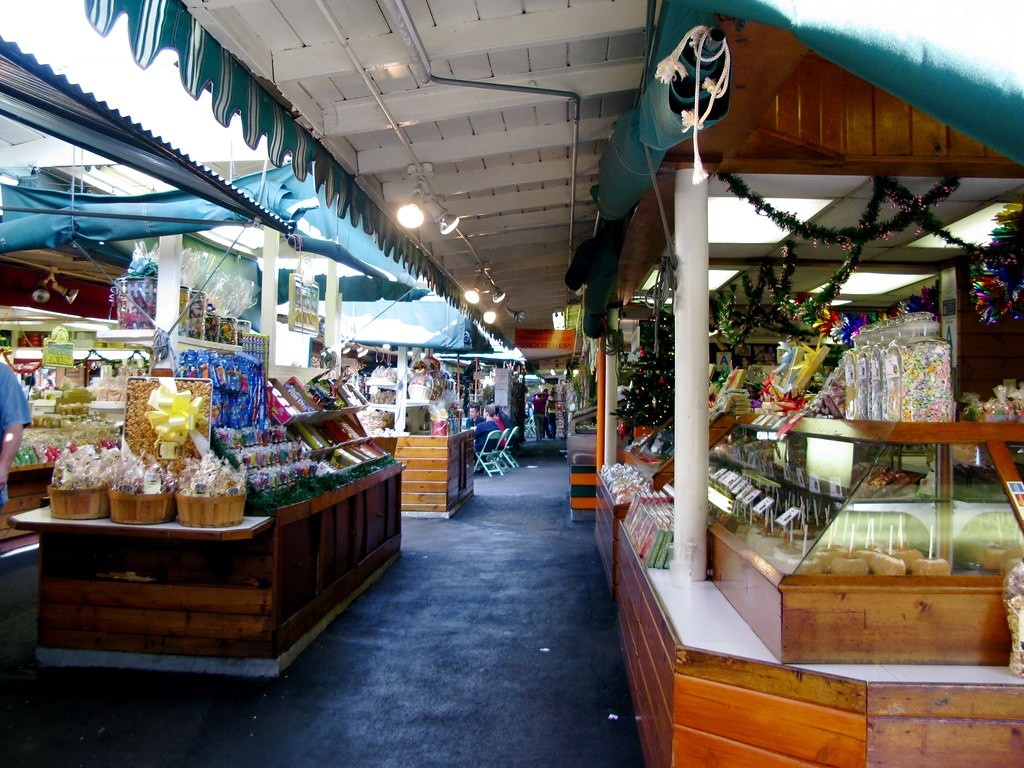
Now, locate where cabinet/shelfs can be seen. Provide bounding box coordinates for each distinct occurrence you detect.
[660,411,1024,664]
[364,346,438,436]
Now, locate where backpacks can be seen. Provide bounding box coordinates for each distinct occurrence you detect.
[547,399,557,410]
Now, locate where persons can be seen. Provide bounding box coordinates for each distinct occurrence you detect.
[525,387,557,442]
[466,403,514,474]
[0,362,32,512]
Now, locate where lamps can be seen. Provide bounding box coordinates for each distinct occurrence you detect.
[482,296,525,324]
[30,266,80,305]
[463,262,505,303]
[552,307,565,330]
[342,340,369,359]
[397,183,460,234]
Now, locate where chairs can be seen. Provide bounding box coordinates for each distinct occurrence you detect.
[473,427,520,477]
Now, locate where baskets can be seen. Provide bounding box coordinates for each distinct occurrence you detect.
[47,481,109,520]
[105,486,176,524]
[175,489,247,528]
[407,371,435,400]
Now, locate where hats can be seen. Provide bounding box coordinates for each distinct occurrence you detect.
[550,387,555,396]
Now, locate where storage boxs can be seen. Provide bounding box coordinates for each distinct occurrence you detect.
[267,377,371,450]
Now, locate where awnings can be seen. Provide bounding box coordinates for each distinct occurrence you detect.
[0,37,292,253]
